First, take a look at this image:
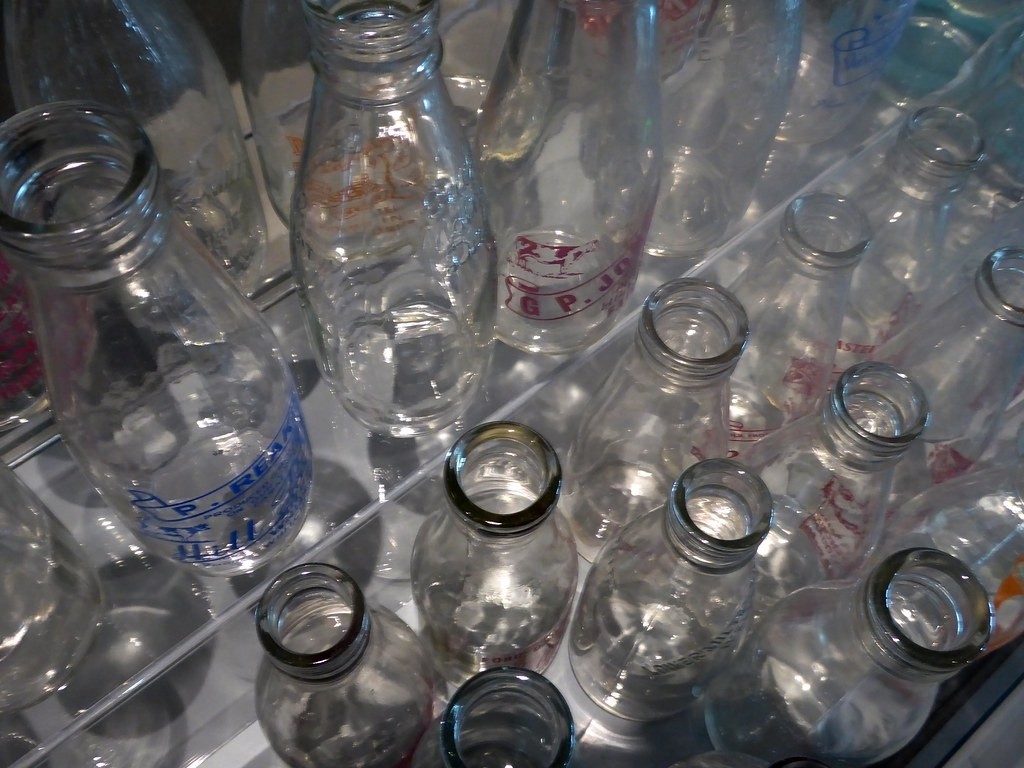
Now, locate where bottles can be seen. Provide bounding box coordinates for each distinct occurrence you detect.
[0,0,1024,768]
